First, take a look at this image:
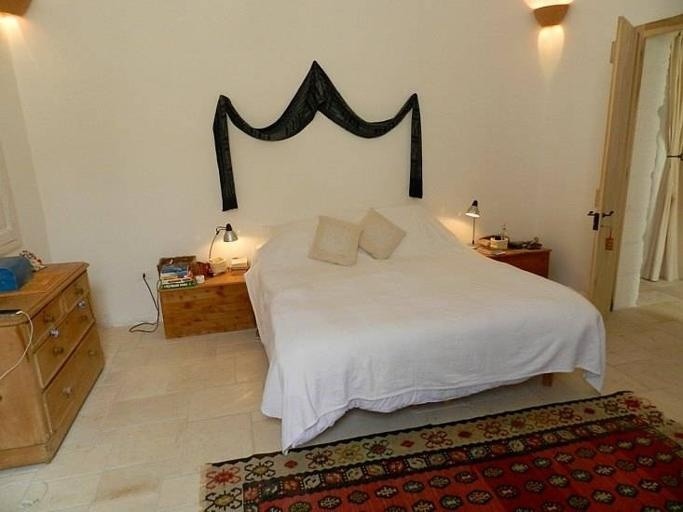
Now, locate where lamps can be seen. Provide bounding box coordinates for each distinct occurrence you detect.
[208,223,238,261]
[465,199,479,250]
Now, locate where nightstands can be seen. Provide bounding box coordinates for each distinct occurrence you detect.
[157,268,258,338]
[467,243,552,280]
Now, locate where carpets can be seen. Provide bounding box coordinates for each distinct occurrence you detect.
[197,390,682,512]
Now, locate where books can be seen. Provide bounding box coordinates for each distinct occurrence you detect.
[160,264,196,289]
[230,256,248,274]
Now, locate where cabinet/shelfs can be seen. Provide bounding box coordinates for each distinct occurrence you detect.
[0,261,105,469]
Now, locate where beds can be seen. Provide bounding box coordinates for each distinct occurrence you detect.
[247,205,597,432]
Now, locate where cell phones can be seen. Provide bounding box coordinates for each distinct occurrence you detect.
[0,309,20,316]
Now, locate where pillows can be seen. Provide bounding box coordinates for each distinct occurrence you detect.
[307,207,404,266]
